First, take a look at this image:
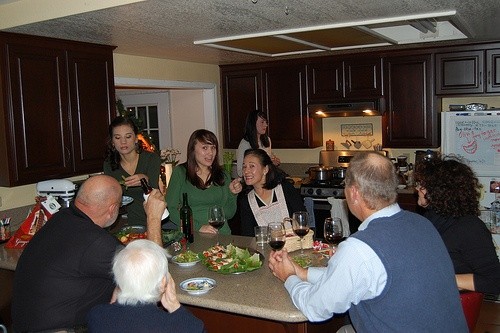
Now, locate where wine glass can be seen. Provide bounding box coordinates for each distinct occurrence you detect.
[208,206,225,247]
[324,217,345,253]
[292,211,310,255]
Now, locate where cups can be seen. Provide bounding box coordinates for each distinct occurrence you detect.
[254,226,269,249]
[268,222,286,252]
[0,224,11,243]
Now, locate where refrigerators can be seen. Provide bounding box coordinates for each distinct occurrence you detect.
[440,111,500,262]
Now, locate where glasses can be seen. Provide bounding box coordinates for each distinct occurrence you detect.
[415,181,426,191]
[340,180,355,187]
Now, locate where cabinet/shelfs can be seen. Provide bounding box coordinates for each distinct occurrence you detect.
[220,60,323,150]
[435,41,500,98]
[0,31,118,188]
[307,51,385,104]
[385,49,441,148]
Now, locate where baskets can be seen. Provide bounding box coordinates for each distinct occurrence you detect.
[272,226,314,253]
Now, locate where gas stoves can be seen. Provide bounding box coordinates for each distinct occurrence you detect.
[300,150,387,199]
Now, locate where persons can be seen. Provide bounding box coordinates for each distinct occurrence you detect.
[268,150,469,333]
[236,110,280,178]
[83,239,205,333]
[102,115,161,234]
[165,129,242,235]
[414,153,500,329]
[227,150,308,238]
[7,175,167,333]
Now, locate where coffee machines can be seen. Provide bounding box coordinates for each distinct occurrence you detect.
[397,156,408,183]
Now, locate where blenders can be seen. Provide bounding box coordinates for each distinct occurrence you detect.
[37,179,79,210]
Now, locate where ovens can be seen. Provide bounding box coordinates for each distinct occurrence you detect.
[300,200,356,242]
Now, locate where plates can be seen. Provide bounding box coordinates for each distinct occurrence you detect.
[121,196,135,206]
[298,254,313,269]
[180,277,217,295]
[171,246,265,275]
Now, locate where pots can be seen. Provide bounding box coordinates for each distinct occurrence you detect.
[305,163,348,182]
[372,144,383,151]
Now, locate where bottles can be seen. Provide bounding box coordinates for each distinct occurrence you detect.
[141,178,171,225]
[325,139,334,151]
[180,192,195,244]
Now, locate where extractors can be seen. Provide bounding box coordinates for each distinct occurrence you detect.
[309,102,381,119]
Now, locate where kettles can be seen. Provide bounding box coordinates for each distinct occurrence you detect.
[231,160,237,179]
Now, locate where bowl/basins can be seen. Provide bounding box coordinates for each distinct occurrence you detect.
[449,103,487,111]
